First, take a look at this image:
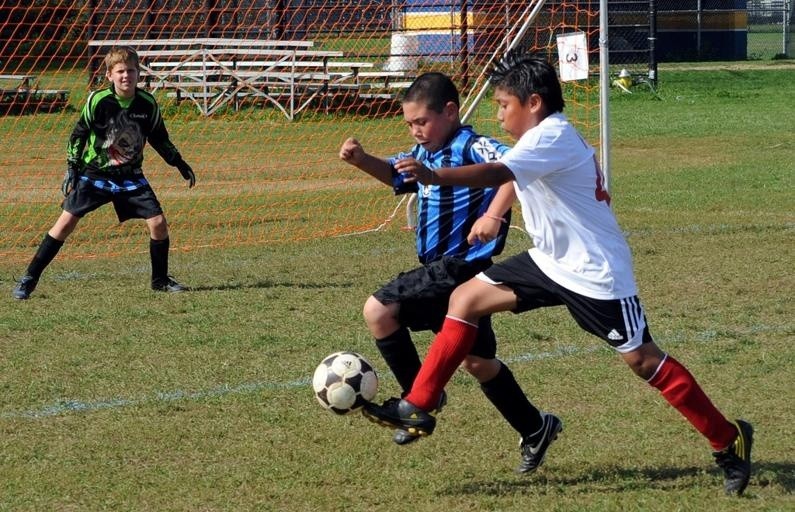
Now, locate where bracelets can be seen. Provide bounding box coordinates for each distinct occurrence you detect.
[430,169,435,186]
[482,212,507,223]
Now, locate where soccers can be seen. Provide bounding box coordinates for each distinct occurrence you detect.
[313,352,379,415]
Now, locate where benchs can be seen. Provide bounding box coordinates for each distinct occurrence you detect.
[86,37,416,124]
[0,73,69,113]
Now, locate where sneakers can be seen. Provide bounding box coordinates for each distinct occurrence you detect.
[12,269,37,299]
[518,410,563,477]
[362,388,447,445]
[711,419,755,497]
[151,275,190,294]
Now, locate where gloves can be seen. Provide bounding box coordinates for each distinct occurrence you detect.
[177,160,195,188]
[62,165,79,197]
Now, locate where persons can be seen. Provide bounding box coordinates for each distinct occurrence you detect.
[361,49,754,500]
[339,72,564,478]
[12,48,196,301]
